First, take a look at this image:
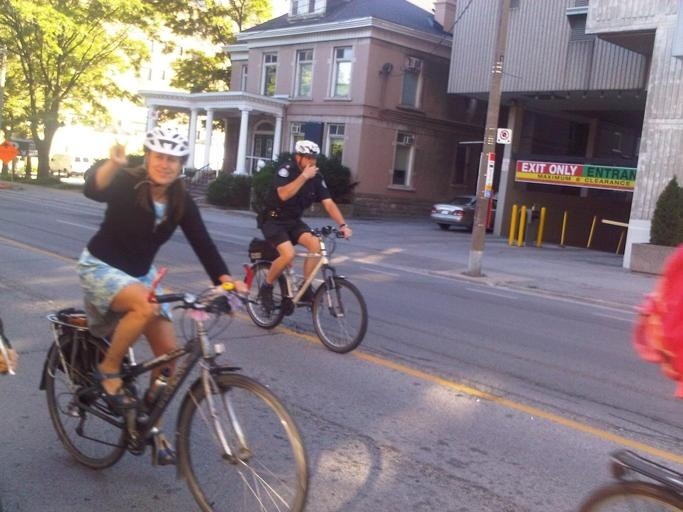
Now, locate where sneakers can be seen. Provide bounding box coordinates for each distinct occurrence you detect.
[260,284,273,310]
[300,282,314,301]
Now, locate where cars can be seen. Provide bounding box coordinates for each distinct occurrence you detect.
[430,193,500,236]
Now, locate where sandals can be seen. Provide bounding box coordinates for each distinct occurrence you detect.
[92,365,140,408]
[152,426,177,465]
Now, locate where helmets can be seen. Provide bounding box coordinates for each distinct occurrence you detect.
[144,127,190,157]
[295,140,320,156]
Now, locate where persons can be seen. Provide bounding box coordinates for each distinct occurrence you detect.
[75,129,250,466]
[258,139,353,311]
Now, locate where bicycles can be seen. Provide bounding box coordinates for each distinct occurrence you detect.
[573,449,683,512]
[239,224,368,355]
[34,279,310,510]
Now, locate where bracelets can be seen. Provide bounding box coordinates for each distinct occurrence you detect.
[339,224,349,229]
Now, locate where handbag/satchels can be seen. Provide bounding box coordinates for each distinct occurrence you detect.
[249,238,273,262]
[57,308,130,374]
